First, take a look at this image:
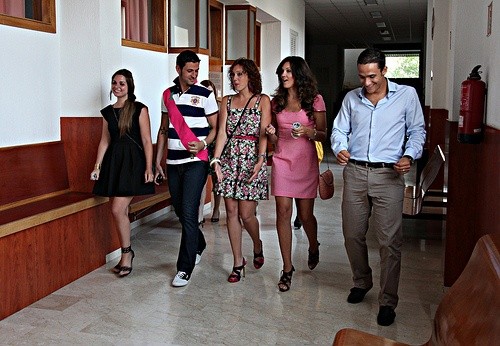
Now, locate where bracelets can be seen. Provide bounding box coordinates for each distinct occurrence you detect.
[210,157,221,168]
[258,153,266,158]
[309,129,317,140]
[94,164,101,169]
[266,132,271,135]
[201,140,207,150]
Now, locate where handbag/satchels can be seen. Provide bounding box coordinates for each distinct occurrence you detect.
[152,167,163,183]
[319,170,334,200]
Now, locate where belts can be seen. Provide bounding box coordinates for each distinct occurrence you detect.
[348,159,394,168]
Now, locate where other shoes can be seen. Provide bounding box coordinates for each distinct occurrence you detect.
[347,283,373,303]
[377,305,396,326]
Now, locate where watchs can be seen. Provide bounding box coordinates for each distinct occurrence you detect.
[403,155,415,166]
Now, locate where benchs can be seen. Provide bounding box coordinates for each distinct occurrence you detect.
[129,181,171,220]
[404,144,445,220]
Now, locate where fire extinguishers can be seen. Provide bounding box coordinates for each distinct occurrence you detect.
[456,65,487,144]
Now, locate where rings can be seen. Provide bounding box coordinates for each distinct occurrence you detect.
[151,175,153,183]
[404,172,406,174]
[330,49,426,326]
[341,155,345,159]
[403,169,405,171]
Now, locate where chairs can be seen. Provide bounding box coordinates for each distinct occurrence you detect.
[332,234,500,346]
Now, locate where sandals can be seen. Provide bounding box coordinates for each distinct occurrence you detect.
[113,263,120,273]
[119,245,134,276]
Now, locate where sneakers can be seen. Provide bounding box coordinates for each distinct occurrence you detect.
[194,245,207,264]
[171,264,196,286]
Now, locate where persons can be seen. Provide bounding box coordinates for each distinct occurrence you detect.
[265,56,326,292]
[198,80,221,227]
[210,59,271,282]
[90,69,154,276]
[154,50,218,287]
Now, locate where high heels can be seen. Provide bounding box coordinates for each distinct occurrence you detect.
[294,216,302,229]
[197,218,205,228]
[228,257,247,282]
[211,211,220,222]
[278,265,295,292]
[253,240,264,269]
[308,241,320,270]
